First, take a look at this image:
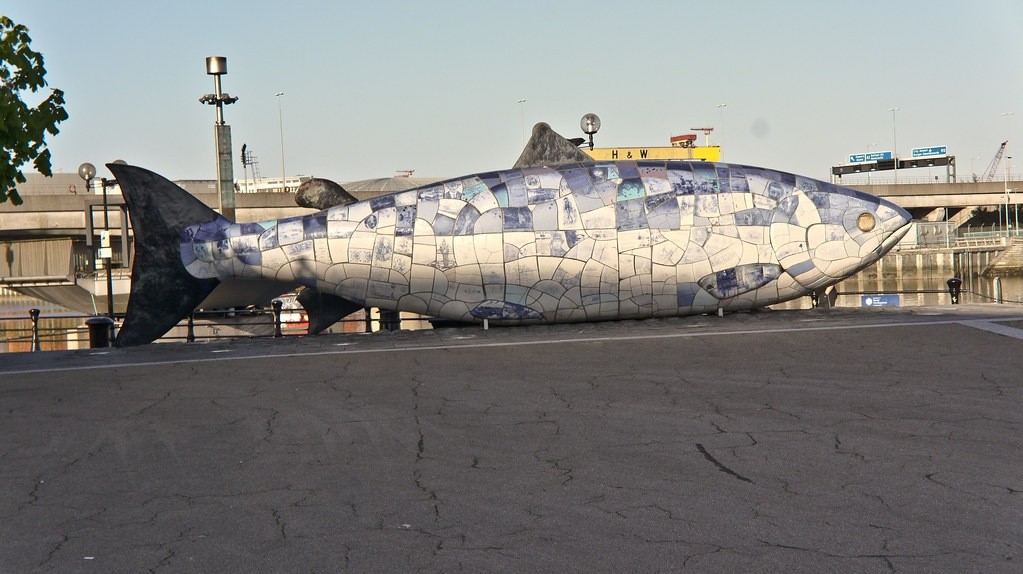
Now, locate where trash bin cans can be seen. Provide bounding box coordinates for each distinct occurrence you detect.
[84,316,114,349]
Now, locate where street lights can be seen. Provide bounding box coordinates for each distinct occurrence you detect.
[972,157,979,182]
[891,108,900,183]
[1000,112,1013,182]
[517,99,526,152]
[867,144,876,154]
[718,103,726,162]
[1004,155,1012,237]
[1008,166,1016,183]
[275,93,286,192]
[999,195,1004,238]
[1015,188,1019,236]
[80,159,128,348]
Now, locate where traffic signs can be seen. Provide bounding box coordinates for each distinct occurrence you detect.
[866,152,892,162]
[912,145,947,157]
[850,154,866,162]
